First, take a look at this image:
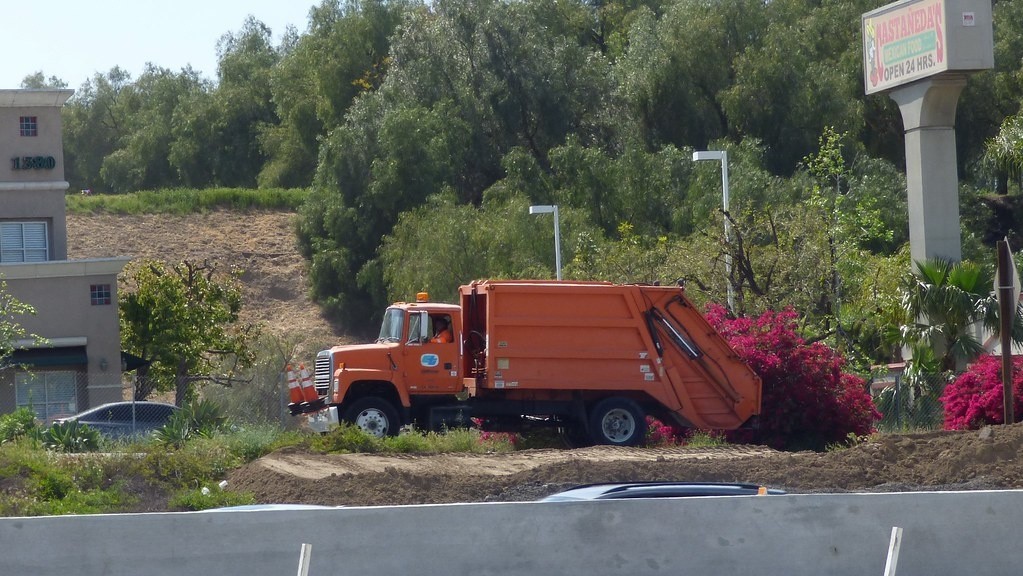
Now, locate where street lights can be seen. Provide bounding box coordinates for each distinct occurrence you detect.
[530,205,562,283]
[692,150,733,312]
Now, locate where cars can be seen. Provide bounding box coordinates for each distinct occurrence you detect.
[537,483,785,501]
[52,400,190,440]
[870,361,909,395]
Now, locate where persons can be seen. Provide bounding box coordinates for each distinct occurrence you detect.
[428,318,452,344]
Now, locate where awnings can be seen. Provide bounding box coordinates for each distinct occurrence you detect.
[120,351,150,376]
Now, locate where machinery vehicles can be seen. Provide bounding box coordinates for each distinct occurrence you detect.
[290,278,762,447]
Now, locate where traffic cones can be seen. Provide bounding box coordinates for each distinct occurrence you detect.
[285,364,327,415]
